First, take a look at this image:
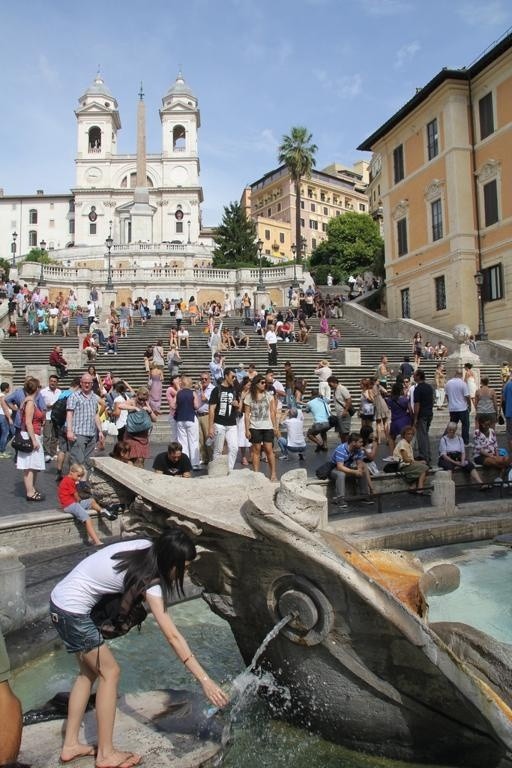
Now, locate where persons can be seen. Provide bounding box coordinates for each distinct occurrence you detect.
[49,527,230,768]
[167,343,181,376]
[173,376,205,471]
[398,356,415,378]
[423,341,436,360]
[434,341,449,359]
[241,292,252,318]
[1,278,85,341]
[221,327,251,351]
[151,260,178,278]
[360,425,379,500]
[385,382,414,456]
[90,285,99,318]
[327,325,341,350]
[392,425,430,496]
[498,379,512,455]
[326,376,352,444]
[208,352,227,386]
[253,285,316,366]
[57,462,118,547]
[164,298,186,330]
[84,300,95,325]
[413,370,435,466]
[153,295,164,321]
[199,300,223,316]
[54,377,82,482]
[39,375,62,463]
[327,273,334,286]
[315,286,346,318]
[472,417,512,489]
[463,362,478,410]
[376,356,394,392]
[152,441,194,479]
[88,365,104,398]
[500,360,511,383]
[237,361,306,482]
[326,432,375,508]
[66,373,105,465]
[439,421,493,491]
[170,327,179,350]
[307,389,332,453]
[207,313,215,332]
[357,379,375,430]
[320,314,331,334]
[103,297,148,356]
[102,339,165,469]
[166,374,181,443]
[370,376,391,446]
[178,326,190,351]
[49,345,69,378]
[0,626,23,768]
[16,378,45,502]
[193,261,213,278]
[402,376,410,401]
[314,359,332,412]
[435,362,446,411]
[188,295,199,327]
[412,331,423,367]
[346,270,379,300]
[4,375,48,466]
[196,372,216,465]
[474,377,499,430]
[0,382,16,460]
[207,317,223,363]
[82,318,107,361]
[469,334,476,354]
[208,367,244,476]
[445,368,474,447]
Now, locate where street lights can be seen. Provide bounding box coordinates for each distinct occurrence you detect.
[105,235,114,284]
[106,236,113,290]
[40,241,46,282]
[11,230,18,267]
[291,243,299,287]
[38,240,46,286]
[257,239,266,291]
[290,241,299,282]
[256,238,265,287]
[11,232,17,269]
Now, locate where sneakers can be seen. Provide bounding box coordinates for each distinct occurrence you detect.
[410,490,417,494]
[336,497,348,508]
[416,491,430,497]
[0,453,12,459]
[279,455,289,461]
[297,452,305,461]
[45,455,52,463]
[360,497,375,505]
[52,455,57,461]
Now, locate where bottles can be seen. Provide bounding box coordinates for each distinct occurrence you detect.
[198,682,240,720]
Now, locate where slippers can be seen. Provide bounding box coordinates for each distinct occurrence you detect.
[58,745,97,765]
[95,751,144,768]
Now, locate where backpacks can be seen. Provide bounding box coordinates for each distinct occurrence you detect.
[50,396,70,430]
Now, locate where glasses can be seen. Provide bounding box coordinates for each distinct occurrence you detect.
[261,381,266,384]
[201,378,208,381]
[231,376,236,379]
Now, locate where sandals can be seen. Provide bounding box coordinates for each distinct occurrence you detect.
[26,491,46,501]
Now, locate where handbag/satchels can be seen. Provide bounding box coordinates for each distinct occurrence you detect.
[333,391,356,417]
[62,317,69,324]
[37,316,45,322]
[126,409,153,433]
[383,460,405,473]
[101,411,118,436]
[171,352,183,366]
[322,400,338,428]
[497,412,506,425]
[141,301,148,311]
[11,399,37,453]
[316,453,356,480]
